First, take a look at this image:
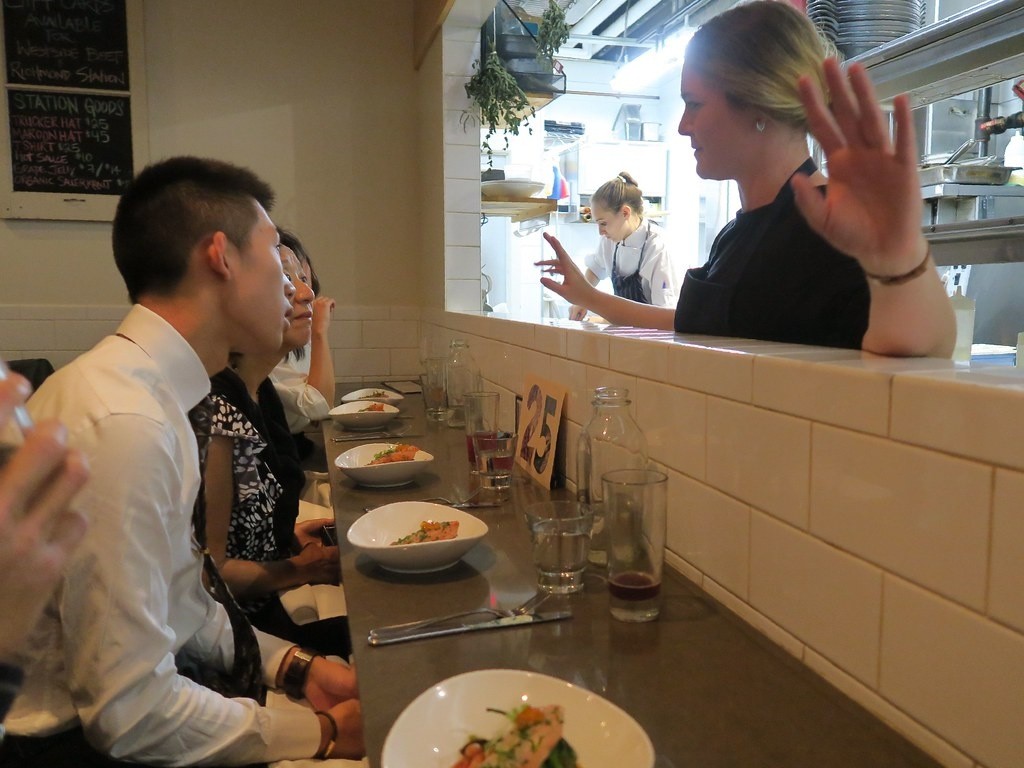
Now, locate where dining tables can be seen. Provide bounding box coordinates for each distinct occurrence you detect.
[320,377,944,768]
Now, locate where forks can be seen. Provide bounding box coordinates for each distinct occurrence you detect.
[330,424,412,440]
[369,593,551,634]
[364,488,480,507]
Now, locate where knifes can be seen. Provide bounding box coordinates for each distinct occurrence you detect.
[366,502,501,511]
[335,433,424,442]
[368,611,573,646]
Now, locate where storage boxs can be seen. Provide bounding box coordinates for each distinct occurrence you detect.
[919,165,1023,187]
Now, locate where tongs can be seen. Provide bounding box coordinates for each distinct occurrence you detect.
[943,138,997,167]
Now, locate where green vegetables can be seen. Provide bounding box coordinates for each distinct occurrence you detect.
[459,703,579,768]
[357,390,386,412]
[396,521,449,544]
[373,444,395,460]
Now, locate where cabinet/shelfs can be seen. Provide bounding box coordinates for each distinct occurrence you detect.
[480,92,558,223]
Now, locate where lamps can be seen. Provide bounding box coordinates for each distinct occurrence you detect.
[611,14,703,93]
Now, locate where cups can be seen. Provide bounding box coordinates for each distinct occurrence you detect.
[602,468,669,623]
[464,392,500,461]
[525,500,588,594]
[417,358,453,421]
[472,432,519,490]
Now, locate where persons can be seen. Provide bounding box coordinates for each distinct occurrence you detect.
[191,229,347,637]
[0,153,367,768]
[0,371,88,728]
[569,172,680,322]
[539,0,959,359]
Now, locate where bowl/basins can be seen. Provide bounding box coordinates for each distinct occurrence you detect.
[328,401,399,430]
[334,443,434,486]
[341,387,403,406]
[380,669,654,768]
[347,501,488,573]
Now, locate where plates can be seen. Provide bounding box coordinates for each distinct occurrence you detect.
[806,0,926,58]
[481,179,544,201]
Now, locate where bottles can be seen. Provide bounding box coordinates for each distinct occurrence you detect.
[576,385,646,568]
[447,335,481,428]
[948,286,976,360]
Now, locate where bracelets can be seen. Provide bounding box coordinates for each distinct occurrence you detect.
[865,245,931,287]
[283,648,323,699]
[315,710,338,759]
